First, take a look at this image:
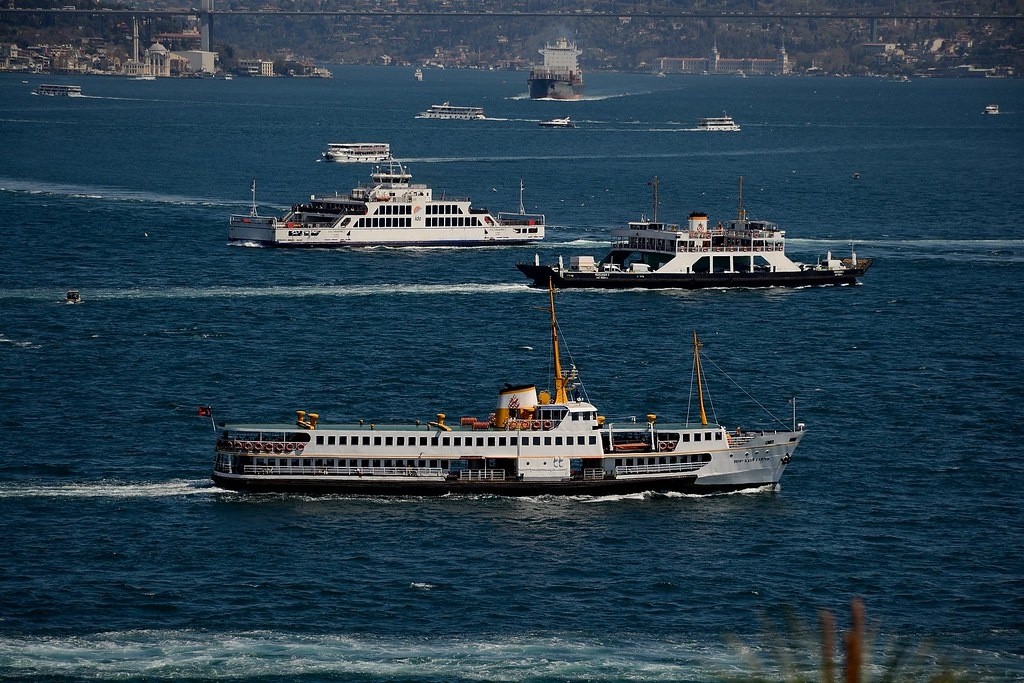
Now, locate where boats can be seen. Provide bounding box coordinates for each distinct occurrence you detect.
[228,154,547,248]
[986,104,1000,116]
[415,101,487,121]
[64,289,82,305]
[198,275,807,494]
[515,172,872,292]
[538,115,574,127]
[696,110,740,133]
[323,142,392,164]
[526,36,585,102]
[37,83,82,99]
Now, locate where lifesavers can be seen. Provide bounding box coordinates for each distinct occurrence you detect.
[656,442,677,449]
[680,244,783,252]
[510,418,554,428]
[228,437,307,452]
